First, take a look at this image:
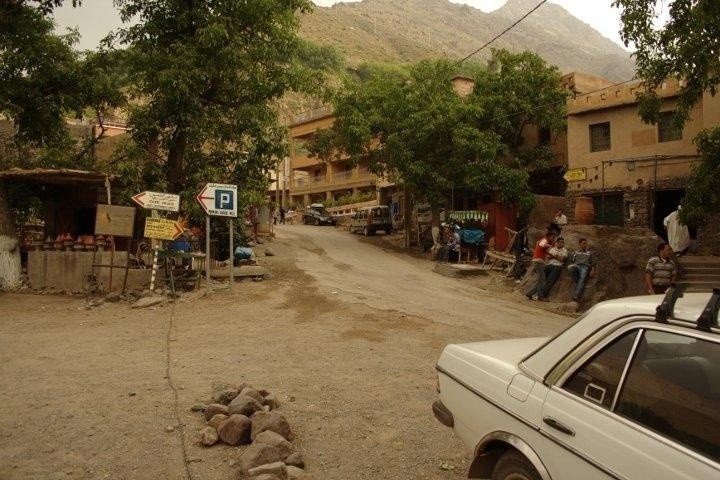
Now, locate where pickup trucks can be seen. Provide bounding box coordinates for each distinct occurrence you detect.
[410,205,445,225]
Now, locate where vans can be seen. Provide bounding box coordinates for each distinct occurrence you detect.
[349,206,392,236]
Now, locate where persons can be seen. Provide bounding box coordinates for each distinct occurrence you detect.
[663,204,691,257]
[272,206,295,225]
[553,209,567,228]
[431,225,460,264]
[526,232,557,302]
[543,238,568,297]
[566,239,596,302]
[645,243,676,294]
[513,223,531,282]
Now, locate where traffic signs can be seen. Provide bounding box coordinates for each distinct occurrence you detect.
[143,218,183,242]
[131,192,180,213]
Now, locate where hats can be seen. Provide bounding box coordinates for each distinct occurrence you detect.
[678,205,682,211]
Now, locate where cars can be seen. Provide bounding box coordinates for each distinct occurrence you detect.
[393,213,404,228]
[434,284,719,480]
[302,212,336,225]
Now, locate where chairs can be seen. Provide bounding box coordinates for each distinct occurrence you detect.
[638,356,716,406]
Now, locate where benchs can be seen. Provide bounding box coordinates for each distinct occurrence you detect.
[456,247,472,264]
